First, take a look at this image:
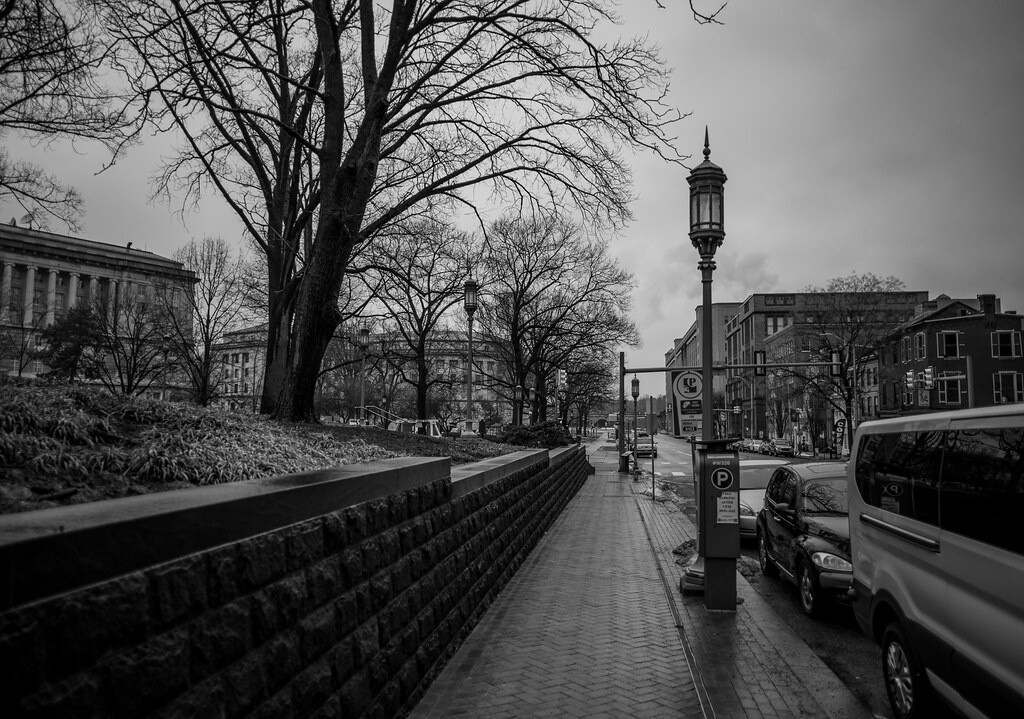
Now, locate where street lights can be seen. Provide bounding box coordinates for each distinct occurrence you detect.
[678,125,729,599]
[358,319,370,426]
[631,372,639,483]
[819,331,858,436]
[460,268,478,439]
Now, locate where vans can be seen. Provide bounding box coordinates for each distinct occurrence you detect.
[846,403,1024,718]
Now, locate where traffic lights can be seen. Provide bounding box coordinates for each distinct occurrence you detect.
[557,369,569,393]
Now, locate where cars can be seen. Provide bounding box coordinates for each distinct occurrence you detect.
[738,458,791,538]
[731,437,794,457]
[755,461,856,616]
[629,436,657,458]
